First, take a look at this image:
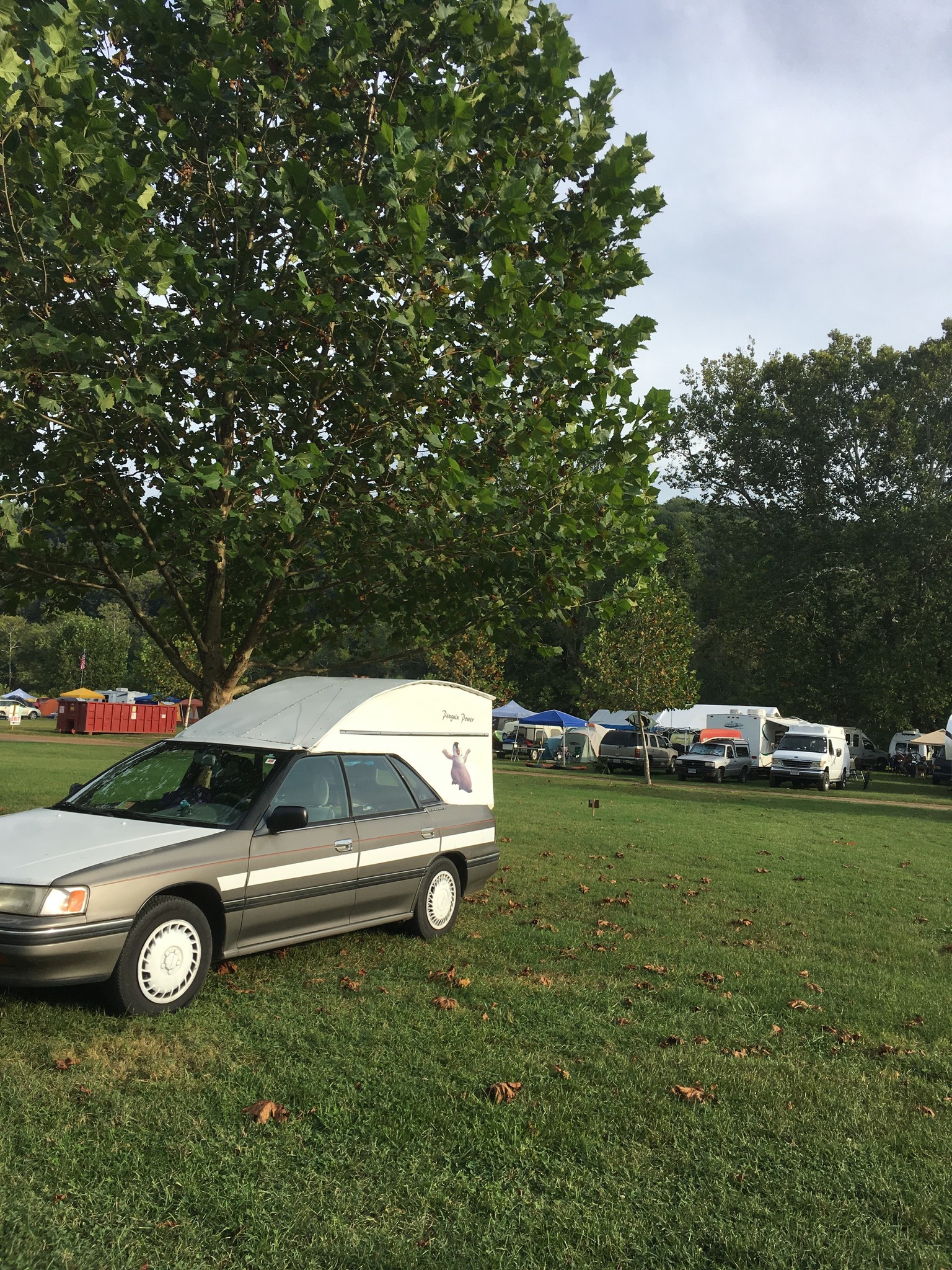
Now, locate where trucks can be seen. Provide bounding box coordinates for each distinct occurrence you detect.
[768,723,851,791]
[837,726,889,771]
[87,687,148,704]
[888,731,934,765]
[674,708,800,784]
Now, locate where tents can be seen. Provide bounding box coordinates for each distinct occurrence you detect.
[492,700,609,767]
[0,688,202,727]
[910,728,946,775]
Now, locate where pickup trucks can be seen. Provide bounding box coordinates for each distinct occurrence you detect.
[27,698,51,709]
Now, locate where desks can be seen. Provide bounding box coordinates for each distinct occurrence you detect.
[512,747,545,764]
[916,765,927,780]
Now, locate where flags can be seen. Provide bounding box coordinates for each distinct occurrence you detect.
[80,653,85,670]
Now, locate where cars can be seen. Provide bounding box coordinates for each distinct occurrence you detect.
[0,675,503,1017]
[0,699,42,721]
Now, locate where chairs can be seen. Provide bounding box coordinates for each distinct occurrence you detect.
[588,755,611,775]
[553,745,570,766]
[570,750,582,763]
[209,752,335,823]
[888,758,904,777]
[846,755,866,782]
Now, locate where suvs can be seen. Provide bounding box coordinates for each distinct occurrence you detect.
[598,728,678,775]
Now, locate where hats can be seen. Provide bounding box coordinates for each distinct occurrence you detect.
[896,750,901,754]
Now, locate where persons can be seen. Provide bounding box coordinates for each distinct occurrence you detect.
[894,752,911,778]
